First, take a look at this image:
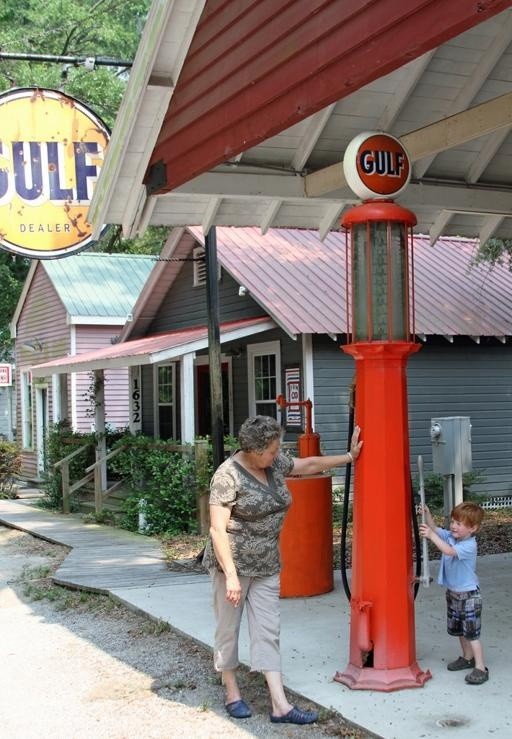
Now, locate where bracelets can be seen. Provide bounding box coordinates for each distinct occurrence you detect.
[347,451,353,461]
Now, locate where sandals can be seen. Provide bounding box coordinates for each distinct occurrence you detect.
[447,656,489,684]
[225,699,251,718]
[269,707,318,724]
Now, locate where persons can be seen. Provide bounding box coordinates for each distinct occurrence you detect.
[200,412,364,726]
[416,500,489,685]
